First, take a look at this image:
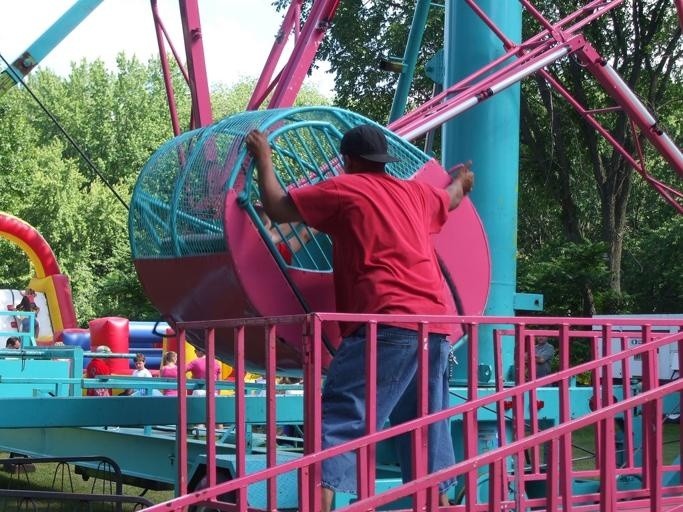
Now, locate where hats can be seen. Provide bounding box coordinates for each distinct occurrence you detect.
[340,125,399,163]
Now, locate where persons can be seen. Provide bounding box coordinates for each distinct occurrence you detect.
[22,307,39,339]
[86,345,304,437]
[16,288,36,311]
[245,124,474,512]
[5,337,20,349]
[252,204,320,266]
[525,336,554,387]
